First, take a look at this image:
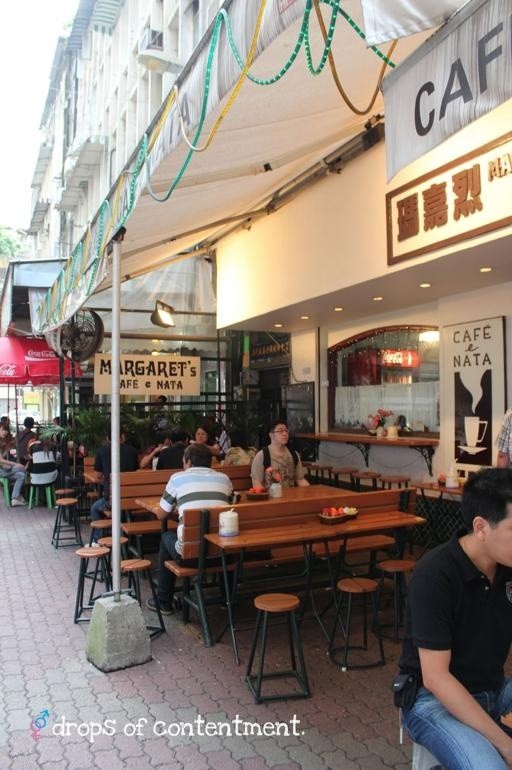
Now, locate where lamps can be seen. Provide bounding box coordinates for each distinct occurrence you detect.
[150,298,179,329]
[133,46,185,77]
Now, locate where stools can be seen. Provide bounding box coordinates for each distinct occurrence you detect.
[409,740,441,769]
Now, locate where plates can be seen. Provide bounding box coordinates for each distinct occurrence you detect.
[457,445,488,455]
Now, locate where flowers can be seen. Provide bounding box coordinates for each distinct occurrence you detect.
[367,407,394,430]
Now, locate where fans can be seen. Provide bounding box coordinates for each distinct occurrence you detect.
[56,308,103,363]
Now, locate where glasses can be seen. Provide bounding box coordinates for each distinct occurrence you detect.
[271,429,289,434]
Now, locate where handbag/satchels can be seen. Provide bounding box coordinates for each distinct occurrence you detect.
[390,673,417,710]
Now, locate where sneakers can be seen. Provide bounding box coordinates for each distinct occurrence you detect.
[145,598,173,615]
[85,542,99,548]
[11,495,28,506]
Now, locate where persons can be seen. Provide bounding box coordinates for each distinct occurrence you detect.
[252,419,315,490]
[491,404,512,470]
[145,443,240,613]
[68,416,84,435]
[141,423,257,467]
[396,469,512,769]
[0,416,67,507]
[86,424,138,551]
[154,395,170,415]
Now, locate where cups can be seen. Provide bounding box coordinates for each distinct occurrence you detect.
[464,416,489,446]
